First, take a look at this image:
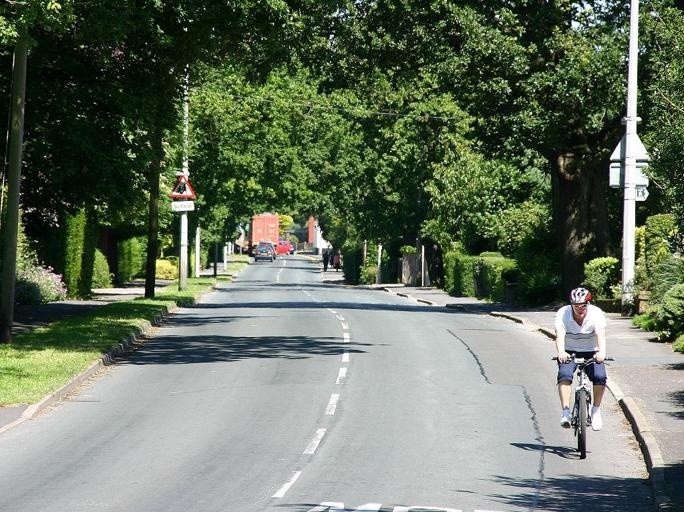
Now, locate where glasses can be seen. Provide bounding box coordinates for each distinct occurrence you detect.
[572,305,587,310]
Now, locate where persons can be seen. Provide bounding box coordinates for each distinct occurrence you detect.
[321,250,330,271]
[553,287,607,432]
[332,253,342,272]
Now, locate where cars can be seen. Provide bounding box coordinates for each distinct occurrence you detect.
[249,240,294,263]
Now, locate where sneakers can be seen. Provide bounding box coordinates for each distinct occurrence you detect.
[591,408,603,431]
[560,410,572,428]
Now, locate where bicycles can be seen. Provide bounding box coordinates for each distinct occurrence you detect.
[550,353,615,461]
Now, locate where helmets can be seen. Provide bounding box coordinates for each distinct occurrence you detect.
[571,288,593,304]
